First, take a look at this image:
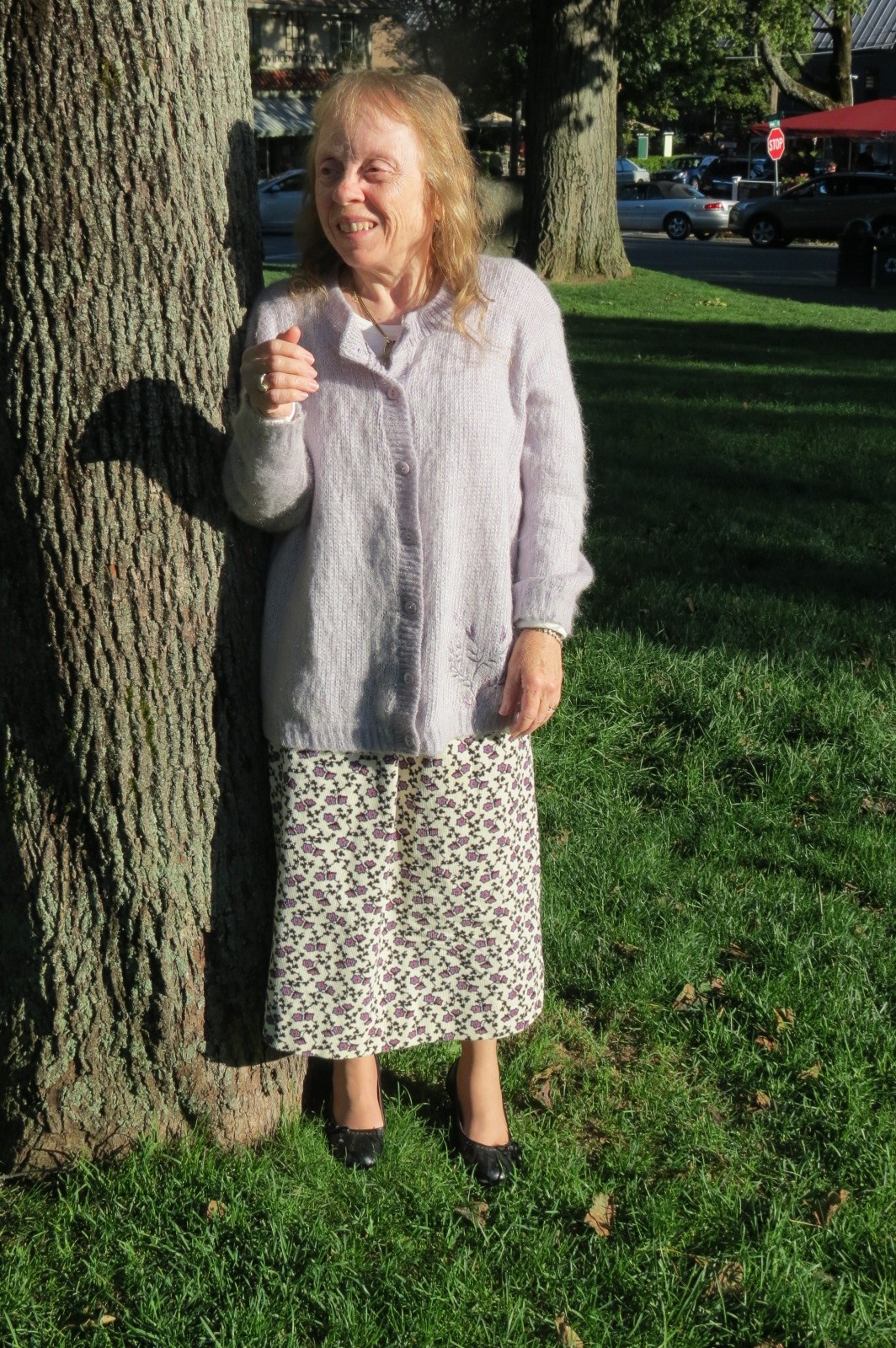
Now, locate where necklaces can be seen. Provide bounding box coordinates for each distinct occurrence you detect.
[348,267,435,360]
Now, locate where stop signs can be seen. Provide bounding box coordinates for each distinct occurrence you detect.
[767,127,785,161]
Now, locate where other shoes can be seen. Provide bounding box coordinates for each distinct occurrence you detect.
[446,1057,516,1179]
[330,1071,387,1166]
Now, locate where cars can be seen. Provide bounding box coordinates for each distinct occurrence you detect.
[649,155,769,197]
[616,180,741,241]
[257,168,308,235]
[616,158,652,191]
[727,170,896,249]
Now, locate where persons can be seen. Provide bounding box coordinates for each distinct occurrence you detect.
[223,64,594,1192]
[765,148,836,173]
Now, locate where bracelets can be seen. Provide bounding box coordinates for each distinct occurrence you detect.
[527,625,564,649]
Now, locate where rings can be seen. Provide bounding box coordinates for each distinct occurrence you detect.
[256,373,270,393]
[547,706,558,712]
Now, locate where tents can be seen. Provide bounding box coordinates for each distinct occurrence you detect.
[747,98,896,195]
